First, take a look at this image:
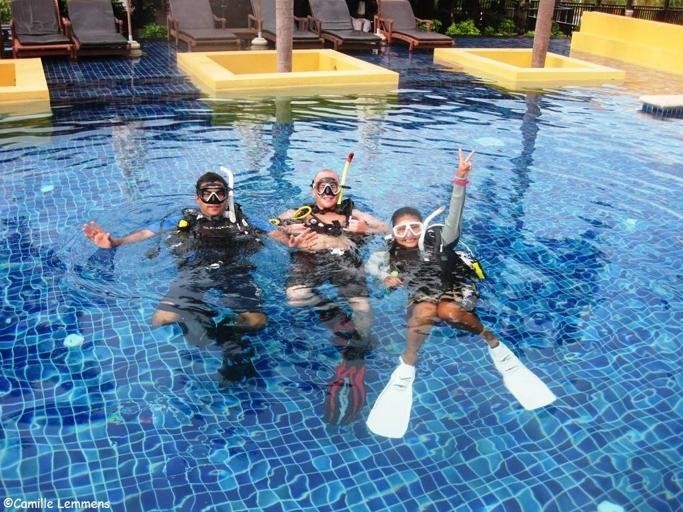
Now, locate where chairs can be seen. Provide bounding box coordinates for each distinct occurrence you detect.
[163,0,455,54]
[9,0,131,63]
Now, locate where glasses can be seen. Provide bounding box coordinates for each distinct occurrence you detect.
[198,183,227,203]
[316,177,340,195]
[393,222,423,237]
